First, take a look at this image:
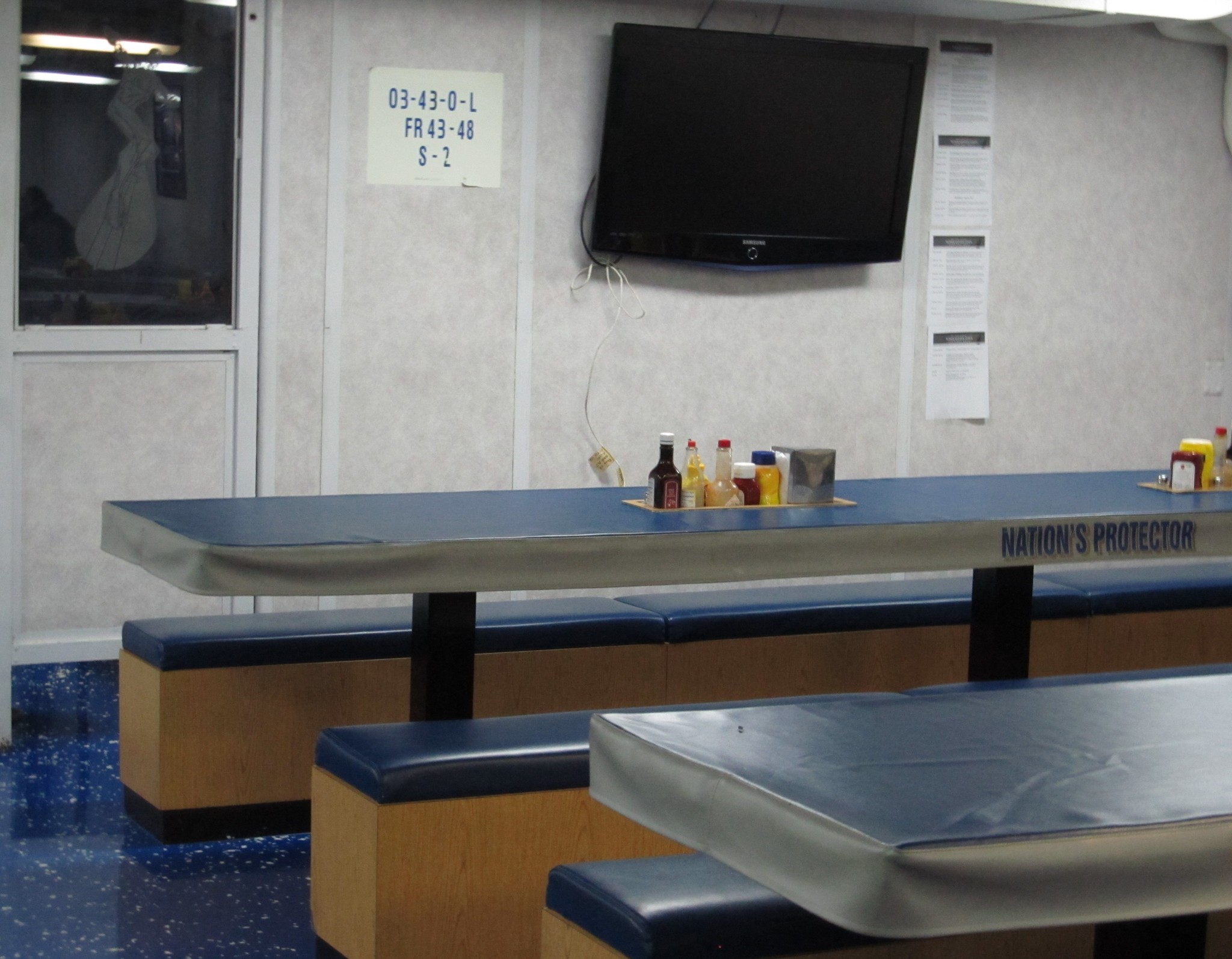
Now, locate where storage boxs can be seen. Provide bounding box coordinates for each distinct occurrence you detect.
[769,445,835,505]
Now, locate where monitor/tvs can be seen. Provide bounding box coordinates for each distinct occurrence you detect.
[586,20,930,269]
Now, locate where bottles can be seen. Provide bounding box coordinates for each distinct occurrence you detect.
[1167,427,1232,488]
[646,432,782,507]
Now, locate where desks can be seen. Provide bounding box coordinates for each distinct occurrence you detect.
[95,460,1231,958]
